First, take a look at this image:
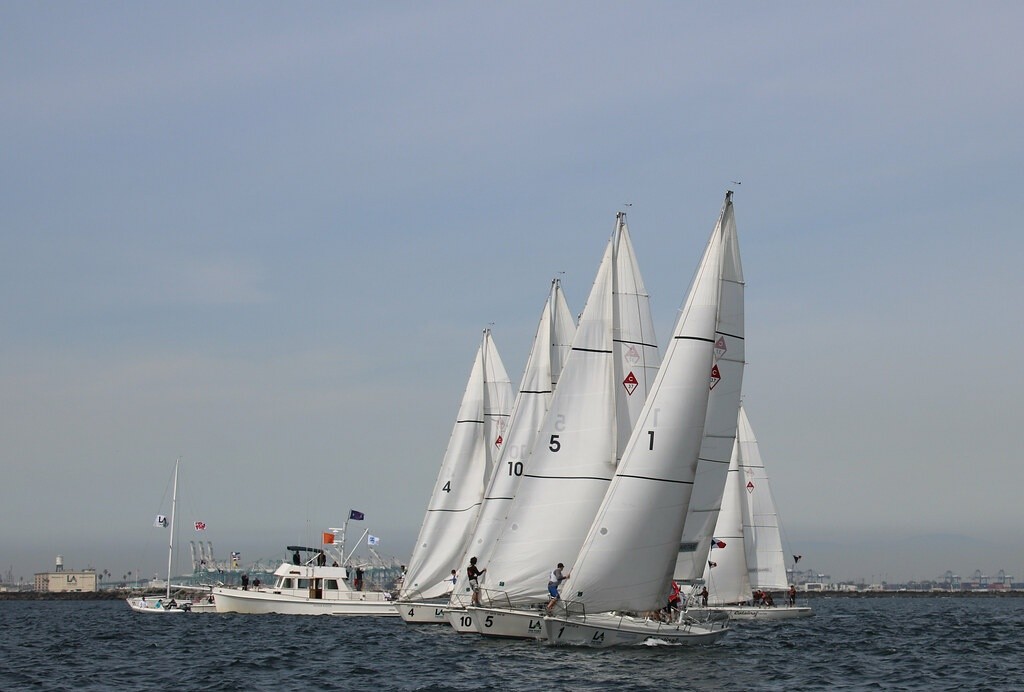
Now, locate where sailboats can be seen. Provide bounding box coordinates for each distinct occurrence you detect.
[385,190,812,646]
[125,459,192,613]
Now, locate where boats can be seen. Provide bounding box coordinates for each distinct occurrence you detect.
[211,546,399,616]
[182,597,216,613]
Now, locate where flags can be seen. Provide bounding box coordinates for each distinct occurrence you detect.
[322,532,335,545]
[154,515,169,528]
[708,561,717,568]
[194,521,206,531]
[710,538,726,550]
[350,509,364,520]
[367,535,380,546]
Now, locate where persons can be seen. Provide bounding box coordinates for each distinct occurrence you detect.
[399,566,408,588]
[752,590,773,607]
[241,574,248,590]
[156,600,162,608]
[332,561,338,567]
[285,579,290,588]
[789,585,796,608]
[451,569,457,585]
[140,597,146,608]
[356,566,364,591]
[467,557,486,607]
[253,578,261,592]
[697,586,709,607]
[208,596,214,604]
[654,579,680,623]
[293,551,300,565]
[317,550,326,566]
[545,563,570,615]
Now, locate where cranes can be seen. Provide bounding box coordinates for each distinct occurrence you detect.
[187,541,404,586]
